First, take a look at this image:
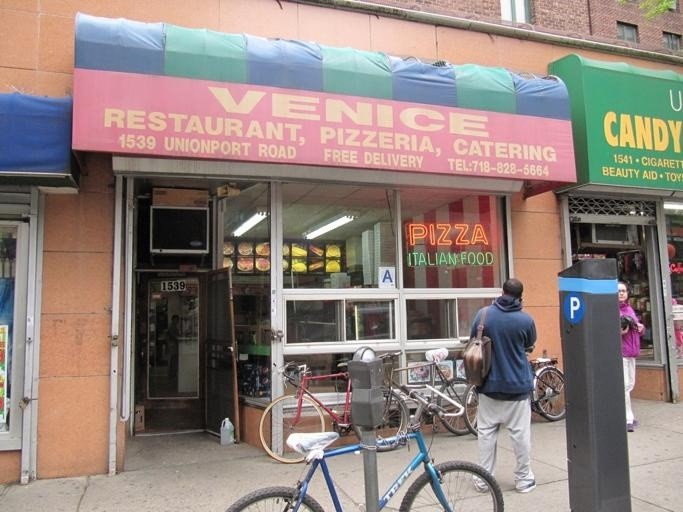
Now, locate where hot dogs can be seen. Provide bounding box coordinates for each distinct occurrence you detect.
[308,261,324,272]
[309,245,323,256]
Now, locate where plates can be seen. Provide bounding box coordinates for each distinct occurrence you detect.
[223,241,270,272]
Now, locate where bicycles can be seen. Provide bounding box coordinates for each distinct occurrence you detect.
[345,348,480,444]
[222,378,507,512]
[459,338,567,436]
[259,360,410,464]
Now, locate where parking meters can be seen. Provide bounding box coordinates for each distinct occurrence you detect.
[347,346,385,512]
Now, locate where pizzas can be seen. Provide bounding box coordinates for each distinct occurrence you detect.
[223,241,270,272]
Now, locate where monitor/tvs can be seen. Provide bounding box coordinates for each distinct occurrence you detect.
[150,206,210,254]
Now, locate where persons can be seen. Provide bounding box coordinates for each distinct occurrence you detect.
[165,315,179,377]
[617,282,646,432]
[471,279,537,493]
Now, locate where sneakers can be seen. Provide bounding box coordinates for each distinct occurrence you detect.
[627,420,637,432]
[472,479,489,493]
[516,479,536,494]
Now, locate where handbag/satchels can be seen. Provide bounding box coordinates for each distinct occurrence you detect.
[463,306,492,387]
